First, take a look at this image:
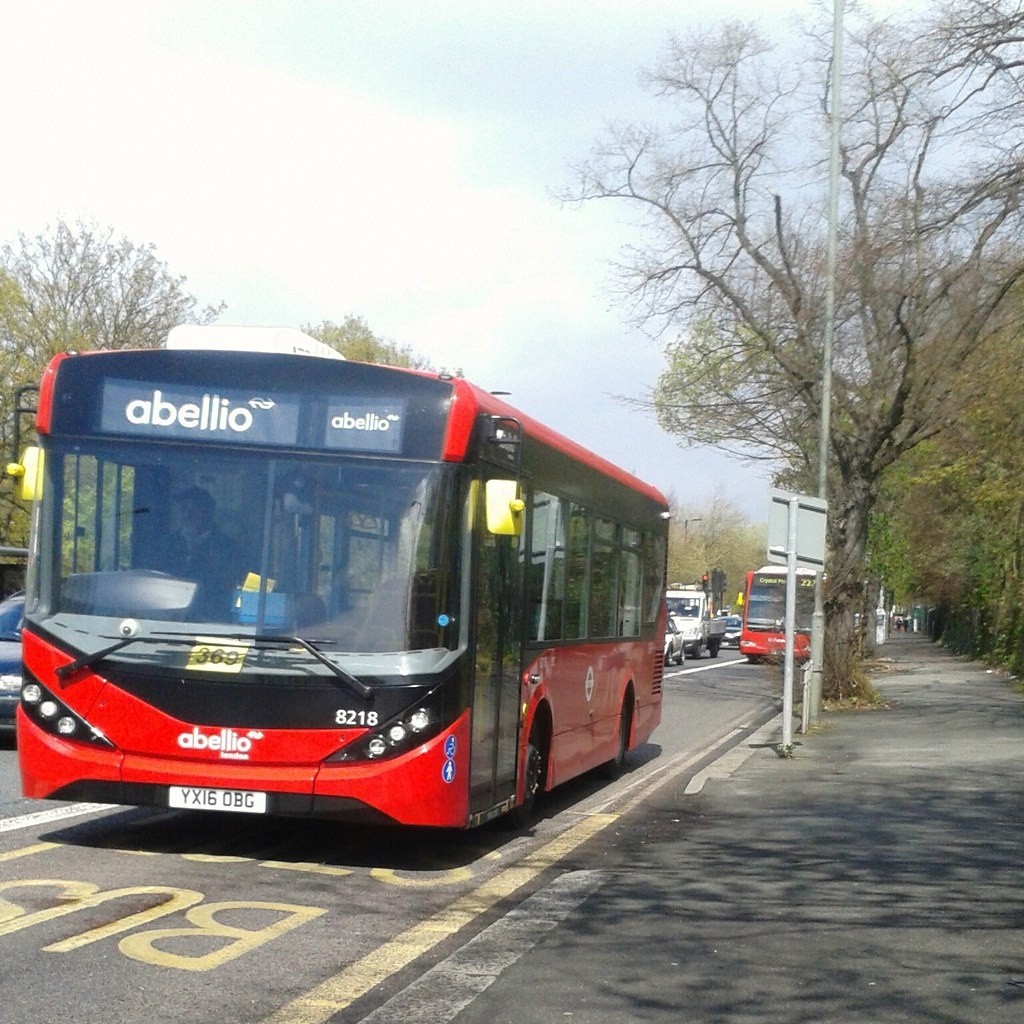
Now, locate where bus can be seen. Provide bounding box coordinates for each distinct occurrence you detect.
[6,320,671,832]
[740,566,827,665]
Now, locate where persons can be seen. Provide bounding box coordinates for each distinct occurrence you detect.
[896,617,909,633]
[143,485,248,625]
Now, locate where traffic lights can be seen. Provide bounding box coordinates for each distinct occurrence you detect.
[720,570,728,593]
[702,574,709,588]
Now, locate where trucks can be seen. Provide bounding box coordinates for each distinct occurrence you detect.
[665,582,726,658]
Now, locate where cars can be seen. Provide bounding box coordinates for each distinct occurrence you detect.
[711,614,741,649]
[0,588,40,731]
[664,613,685,667]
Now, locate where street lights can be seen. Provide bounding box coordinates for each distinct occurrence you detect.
[684,518,702,546]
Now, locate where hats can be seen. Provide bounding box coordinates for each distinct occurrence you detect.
[175,487,216,510]
